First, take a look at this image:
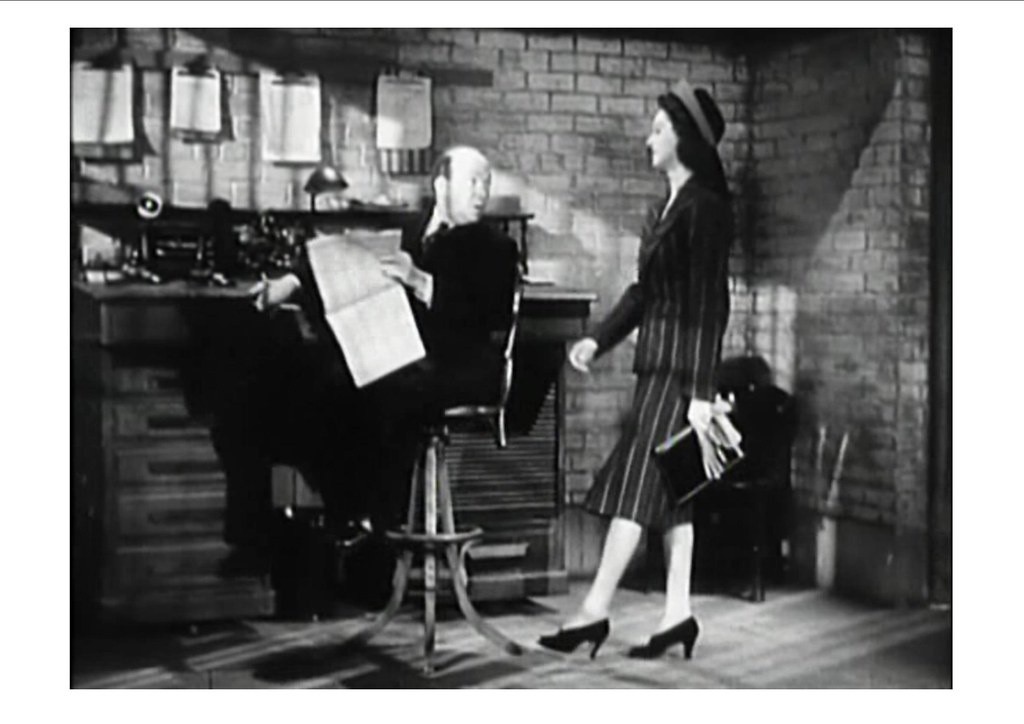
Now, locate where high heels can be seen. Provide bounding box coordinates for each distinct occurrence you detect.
[630,616,698,660]
[539,618,610,658]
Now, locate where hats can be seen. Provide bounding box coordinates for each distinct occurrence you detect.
[657,80,725,156]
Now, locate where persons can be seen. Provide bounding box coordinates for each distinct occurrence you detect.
[532,86,735,664]
[206,145,520,571]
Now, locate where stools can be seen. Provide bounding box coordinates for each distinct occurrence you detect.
[346,270,524,678]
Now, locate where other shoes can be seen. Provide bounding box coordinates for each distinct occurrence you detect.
[330,515,377,551]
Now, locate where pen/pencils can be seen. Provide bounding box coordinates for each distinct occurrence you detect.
[258,270,269,313]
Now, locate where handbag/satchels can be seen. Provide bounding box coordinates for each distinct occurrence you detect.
[653,414,745,511]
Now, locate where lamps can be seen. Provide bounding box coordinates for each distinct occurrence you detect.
[303,163,351,212]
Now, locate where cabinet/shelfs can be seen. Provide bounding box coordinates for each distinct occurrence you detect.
[70,277,600,640]
